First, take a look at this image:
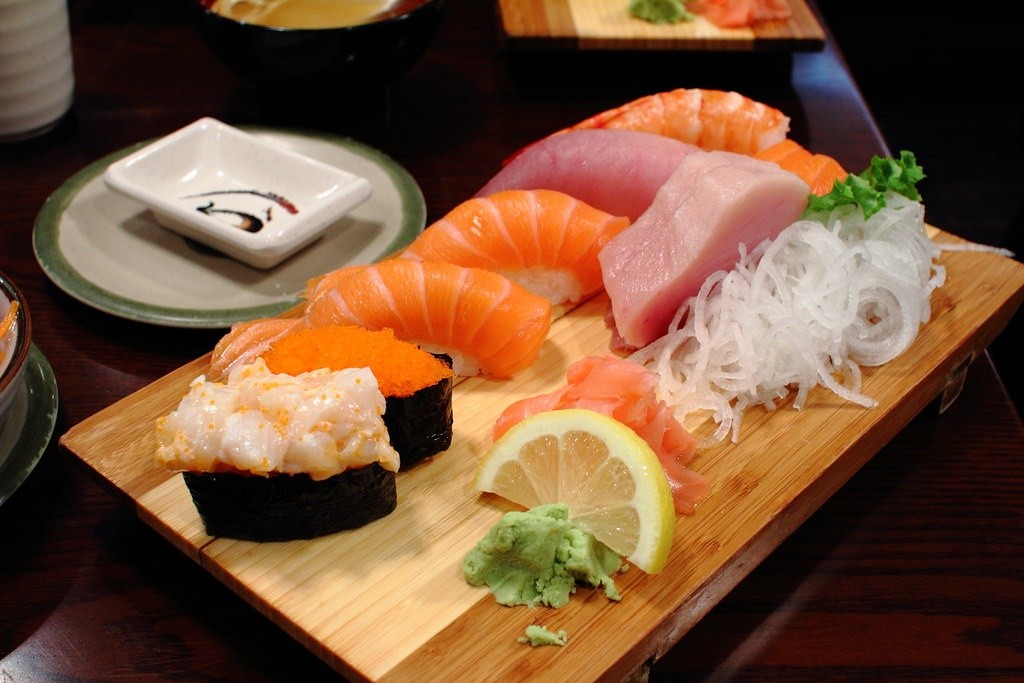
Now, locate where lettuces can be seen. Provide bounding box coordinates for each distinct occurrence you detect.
[804,147,925,220]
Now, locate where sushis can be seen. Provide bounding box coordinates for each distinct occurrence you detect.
[136,88,847,541]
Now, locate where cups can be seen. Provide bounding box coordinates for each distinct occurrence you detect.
[0,0,76,143]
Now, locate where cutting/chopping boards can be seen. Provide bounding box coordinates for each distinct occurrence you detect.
[66,179,1024,683]
[499,0,826,50]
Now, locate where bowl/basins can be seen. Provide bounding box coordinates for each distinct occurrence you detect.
[0,272,31,418]
[194,0,450,92]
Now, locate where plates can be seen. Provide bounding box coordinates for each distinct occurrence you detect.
[0,337,58,507]
[105,116,374,270]
[31,127,428,329]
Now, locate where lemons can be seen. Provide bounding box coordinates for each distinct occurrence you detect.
[472,409,674,574]
